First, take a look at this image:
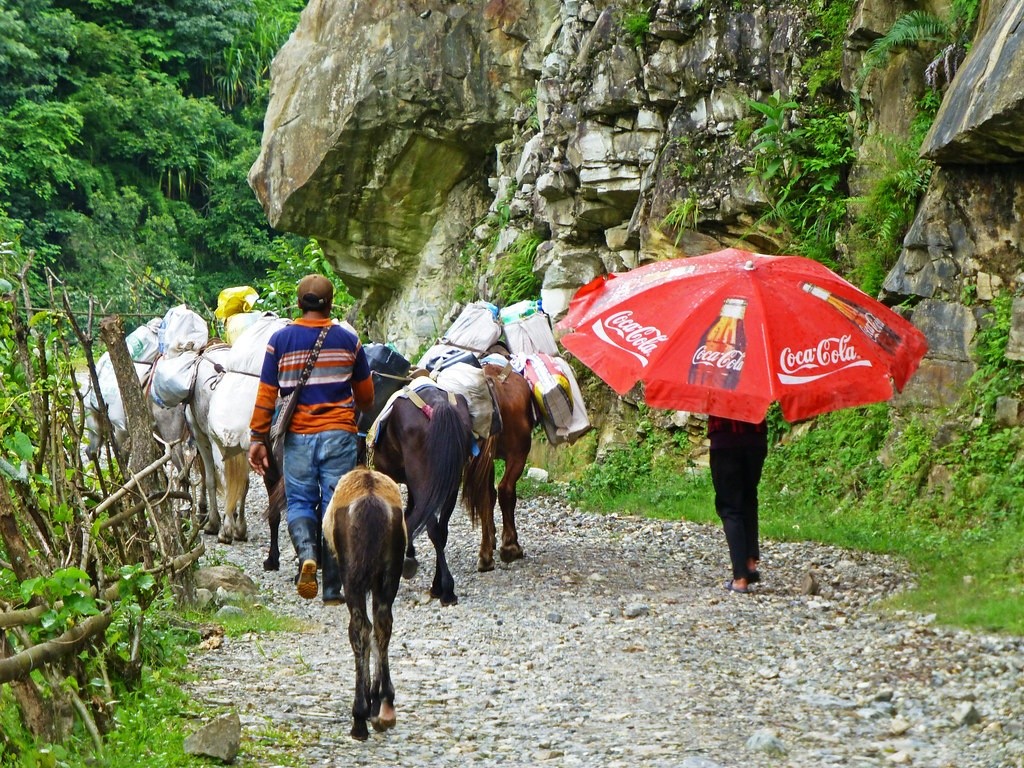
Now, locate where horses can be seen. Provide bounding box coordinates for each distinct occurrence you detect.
[83,335,533,741]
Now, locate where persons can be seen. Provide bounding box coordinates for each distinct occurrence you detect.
[706,413,768,594]
[245,275,374,607]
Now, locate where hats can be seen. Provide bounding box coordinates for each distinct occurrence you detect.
[298,274,333,307]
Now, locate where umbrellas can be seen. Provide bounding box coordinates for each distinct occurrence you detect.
[556,248,930,425]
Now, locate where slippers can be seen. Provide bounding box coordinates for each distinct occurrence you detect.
[747,570,759,582]
[723,578,749,592]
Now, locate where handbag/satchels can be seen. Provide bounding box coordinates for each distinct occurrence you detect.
[269,390,297,438]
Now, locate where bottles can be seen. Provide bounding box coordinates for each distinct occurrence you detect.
[801,280,903,358]
[689,297,749,390]
[497,298,543,324]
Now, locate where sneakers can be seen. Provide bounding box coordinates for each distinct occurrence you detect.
[324,596,347,606]
[297,560,318,598]
